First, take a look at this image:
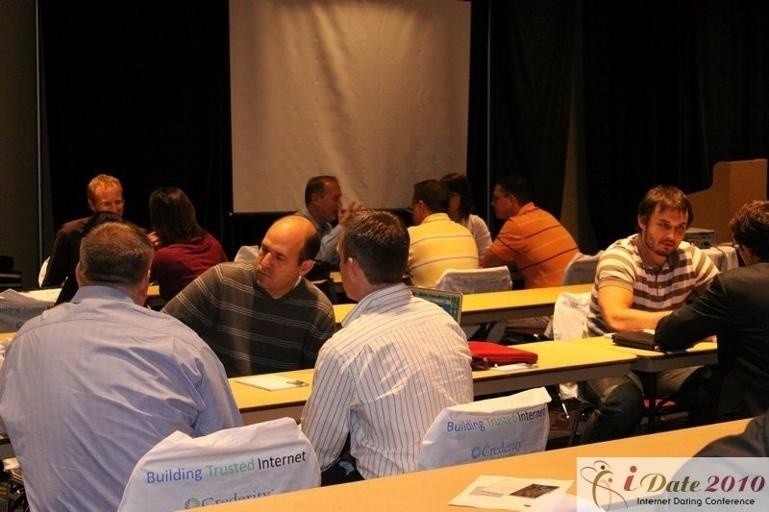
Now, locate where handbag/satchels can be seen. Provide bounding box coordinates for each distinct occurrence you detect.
[468,340,537,367]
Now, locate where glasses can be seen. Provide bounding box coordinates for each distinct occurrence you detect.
[407,205,414,213]
[732,242,742,249]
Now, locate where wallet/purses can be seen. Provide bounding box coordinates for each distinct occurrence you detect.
[612,330,662,352]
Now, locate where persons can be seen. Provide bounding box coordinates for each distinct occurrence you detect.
[1,222,246,511]
[301,212,477,481]
[41,173,769,446]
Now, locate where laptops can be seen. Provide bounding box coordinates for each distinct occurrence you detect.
[411,285,463,325]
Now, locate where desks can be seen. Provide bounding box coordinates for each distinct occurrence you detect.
[0,255,769,510]
